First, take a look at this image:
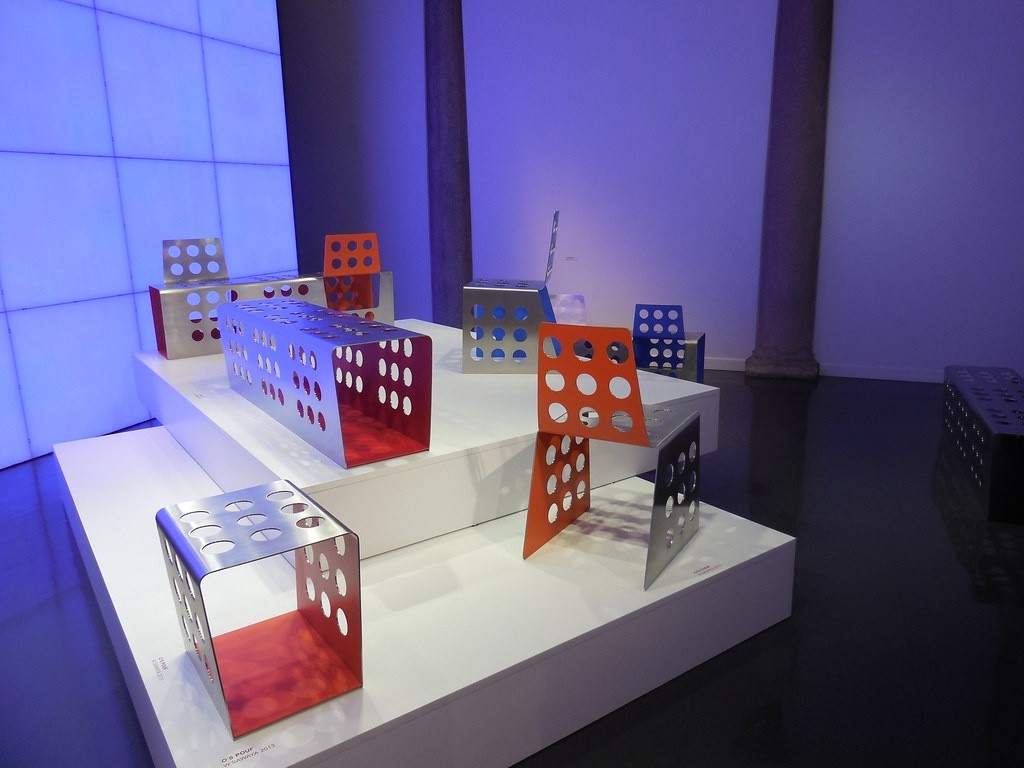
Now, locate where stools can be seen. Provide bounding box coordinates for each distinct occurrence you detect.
[159,481,361,739]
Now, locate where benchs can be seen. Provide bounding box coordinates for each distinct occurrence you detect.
[549,294,705,384]
[943,365,1024,521]
[149,232,394,360]
[216,297,433,470]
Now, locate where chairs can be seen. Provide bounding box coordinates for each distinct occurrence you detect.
[516,322,701,591]
[462,211,560,375]
